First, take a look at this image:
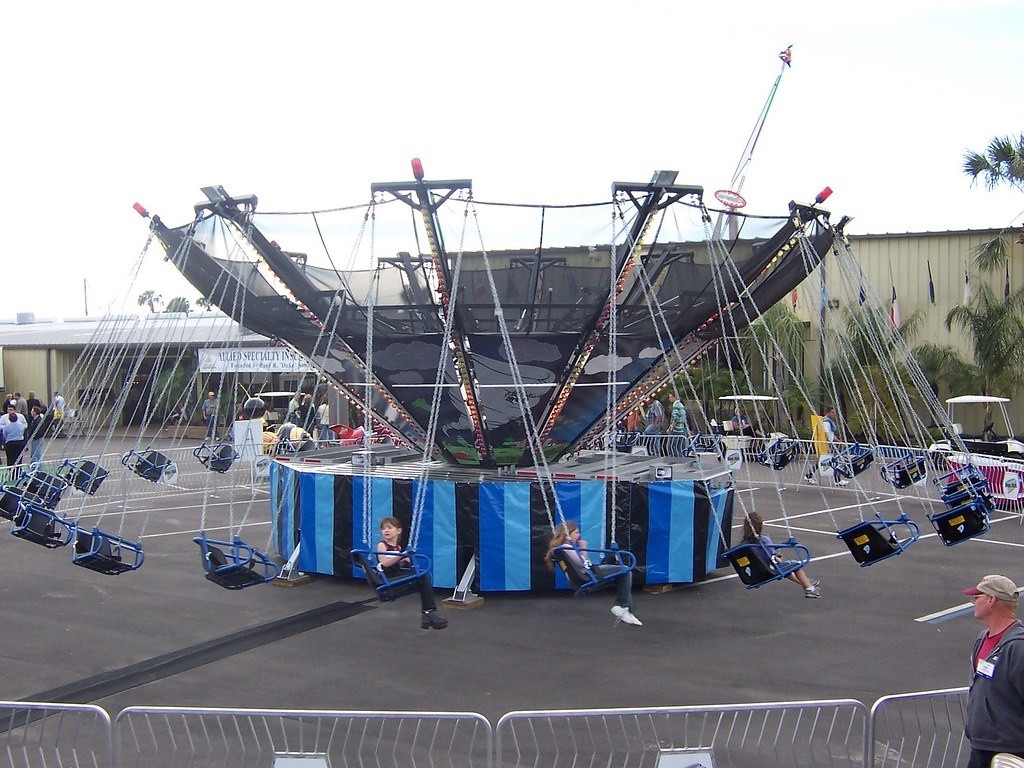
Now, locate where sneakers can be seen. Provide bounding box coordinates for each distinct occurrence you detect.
[804,475,816,485]
[421,609,449,631]
[805,578,823,599]
[835,480,850,486]
[611,604,643,627]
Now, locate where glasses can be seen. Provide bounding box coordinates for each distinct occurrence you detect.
[973,594,986,600]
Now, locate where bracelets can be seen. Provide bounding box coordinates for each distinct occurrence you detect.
[669,425,673,431]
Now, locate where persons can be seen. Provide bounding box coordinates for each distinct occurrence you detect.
[0,391,47,481]
[646,396,665,457]
[202,391,221,441]
[52,392,65,438]
[732,407,771,442]
[804,406,850,486]
[964,574,1024,768]
[737,512,822,599]
[235,392,333,449]
[544,521,643,626]
[666,390,686,457]
[376,517,449,630]
[171,402,191,426]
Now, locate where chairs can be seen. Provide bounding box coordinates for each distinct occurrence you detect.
[951,423,983,442]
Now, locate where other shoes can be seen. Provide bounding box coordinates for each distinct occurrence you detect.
[204,437,221,441]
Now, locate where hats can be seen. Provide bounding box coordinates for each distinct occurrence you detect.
[962,574,1020,601]
